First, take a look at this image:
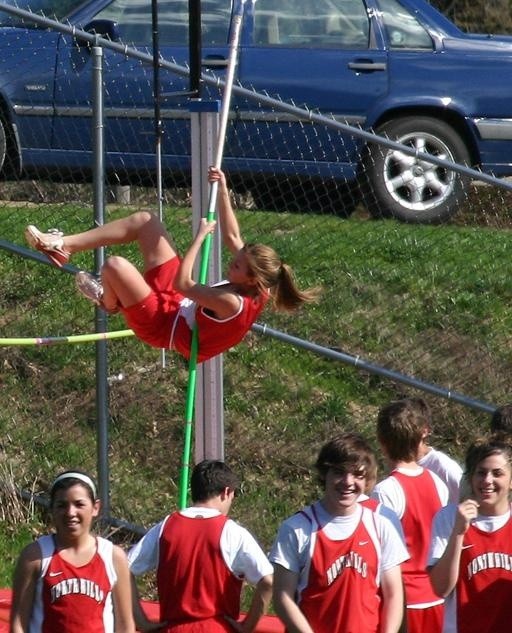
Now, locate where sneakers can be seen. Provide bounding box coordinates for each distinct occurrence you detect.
[25,224,72,268]
[73,268,122,316]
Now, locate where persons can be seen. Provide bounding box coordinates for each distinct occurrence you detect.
[8,470,137,633]
[24,164,324,362]
[425,439,512,632]
[128,460,275,633]
[353,488,403,539]
[405,396,467,632]
[456,401,512,502]
[374,402,453,632]
[266,437,412,632]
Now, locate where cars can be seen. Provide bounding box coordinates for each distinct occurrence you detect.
[0,0,512,226]
